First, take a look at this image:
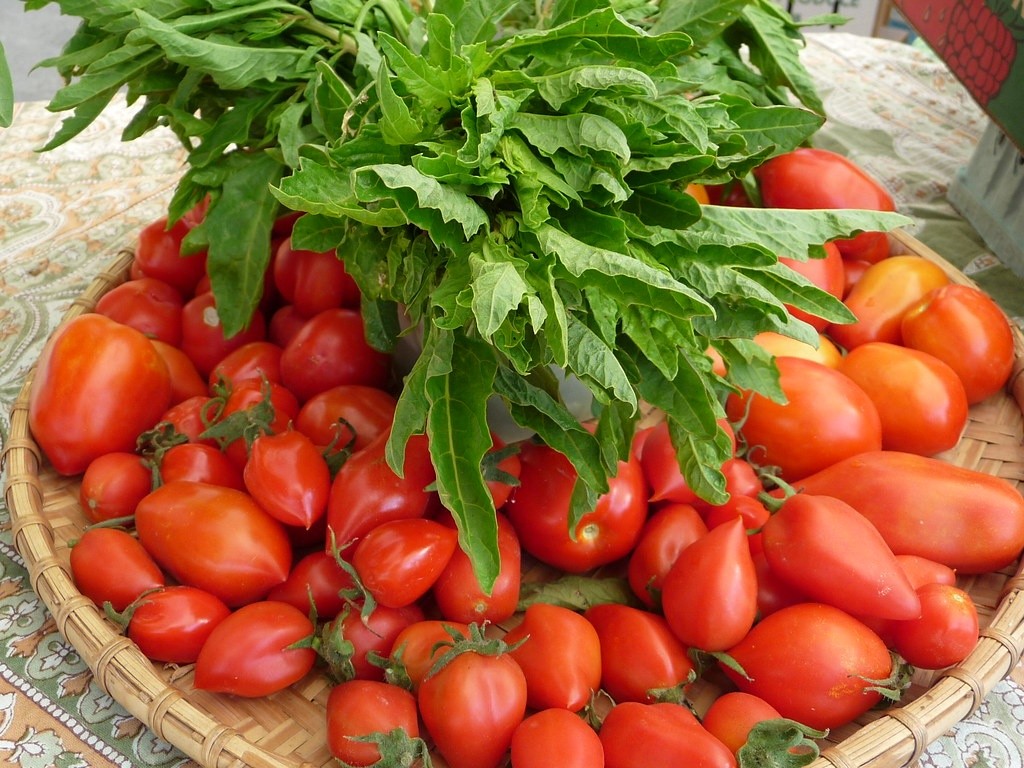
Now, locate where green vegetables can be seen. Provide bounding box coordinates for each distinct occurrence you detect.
[1,0,919,599]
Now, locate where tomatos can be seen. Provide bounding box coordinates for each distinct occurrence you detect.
[0,137,1024,768]
